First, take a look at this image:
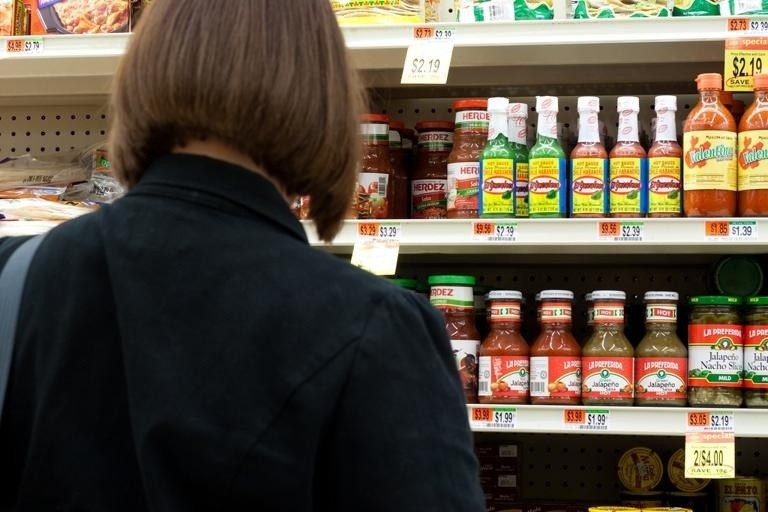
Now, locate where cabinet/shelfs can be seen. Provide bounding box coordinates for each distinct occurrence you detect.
[0,12,766,502]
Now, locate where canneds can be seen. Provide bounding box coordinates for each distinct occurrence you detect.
[718,474,768,512]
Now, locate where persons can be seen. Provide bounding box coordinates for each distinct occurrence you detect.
[0,1,489,512]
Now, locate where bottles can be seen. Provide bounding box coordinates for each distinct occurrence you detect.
[742,296,768,408]
[634,291,688,407]
[401,126,416,219]
[288,195,300,219]
[528,290,581,405]
[507,102,529,219]
[479,97,516,218]
[446,99,489,219]
[477,290,528,404]
[681,72,737,217]
[646,95,683,218]
[410,120,454,219]
[427,275,480,404]
[578,293,592,350]
[471,286,485,343]
[608,95,646,218]
[719,90,731,115]
[580,290,635,405]
[650,117,657,148]
[299,195,309,219]
[527,294,541,350]
[687,296,743,408]
[638,120,642,147]
[345,113,394,219]
[569,96,608,218]
[527,96,566,218]
[415,283,430,302]
[556,122,562,148]
[386,279,418,292]
[476,294,489,344]
[388,119,407,219]
[597,119,604,148]
[737,74,767,216]
